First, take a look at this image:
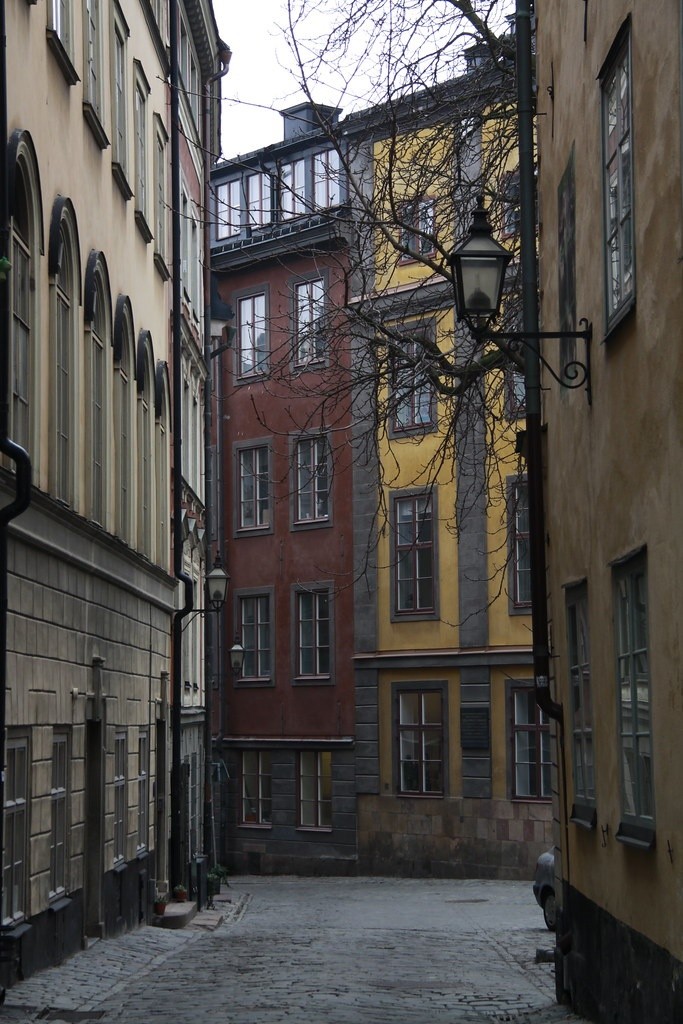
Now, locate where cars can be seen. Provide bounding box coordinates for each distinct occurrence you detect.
[533,844,558,932]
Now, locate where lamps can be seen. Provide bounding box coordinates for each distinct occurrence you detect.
[184,552,229,613]
[443,194,594,403]
[224,634,245,678]
